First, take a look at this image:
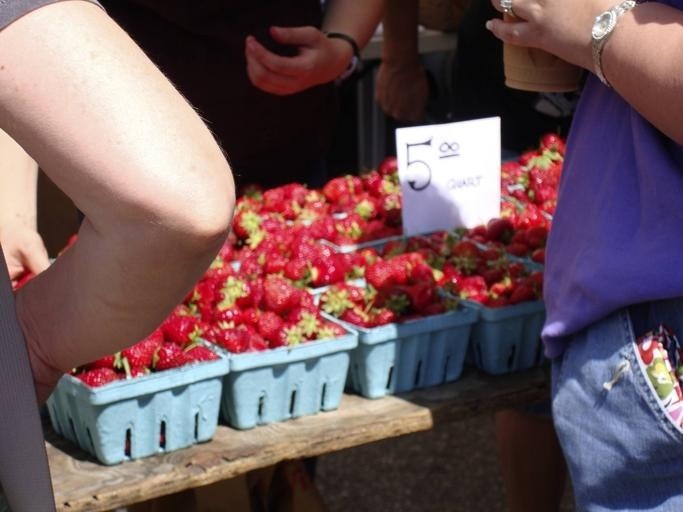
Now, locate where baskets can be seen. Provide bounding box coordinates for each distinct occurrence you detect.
[190,292,360,431]
[45,330,231,468]
[311,284,481,401]
[437,251,553,375]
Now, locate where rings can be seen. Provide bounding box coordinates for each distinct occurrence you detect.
[500,3,519,14]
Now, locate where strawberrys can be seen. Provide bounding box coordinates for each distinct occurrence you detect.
[17,133,566,389]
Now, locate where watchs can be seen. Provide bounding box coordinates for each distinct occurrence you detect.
[589,3,641,85]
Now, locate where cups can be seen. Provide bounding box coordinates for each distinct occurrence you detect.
[503,7,585,91]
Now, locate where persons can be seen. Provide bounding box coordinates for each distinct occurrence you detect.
[0,1,238,512]
[486,1,682,511]
[0,2,384,286]
[374,3,578,155]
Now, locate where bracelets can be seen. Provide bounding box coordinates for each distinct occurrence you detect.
[328,26,361,84]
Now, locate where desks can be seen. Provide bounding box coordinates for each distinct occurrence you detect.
[349,32,459,175]
[42,360,555,512]
